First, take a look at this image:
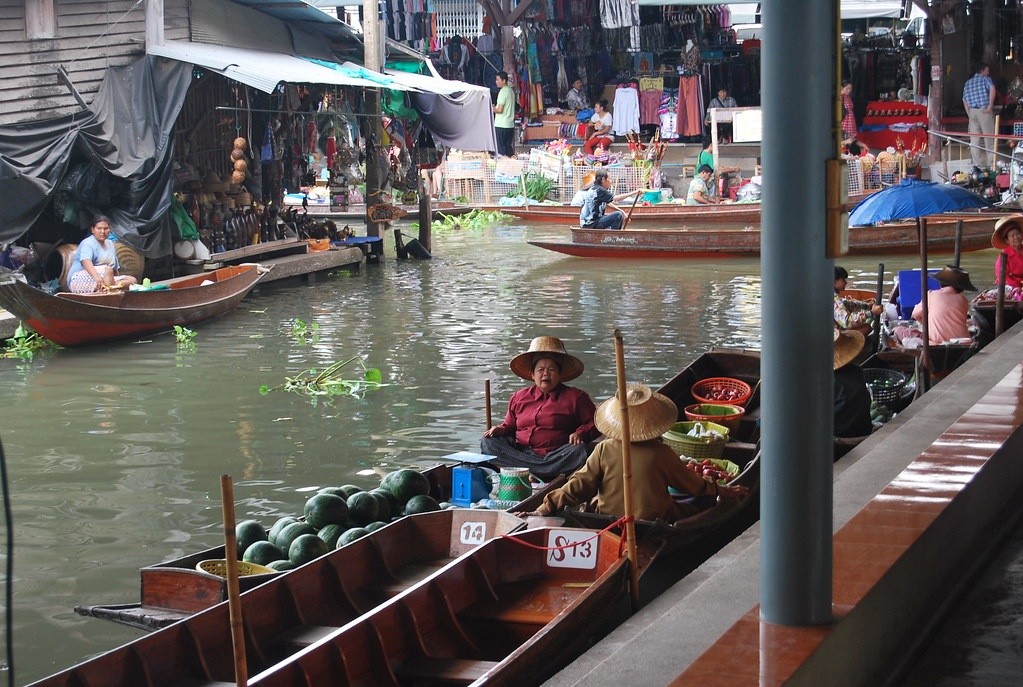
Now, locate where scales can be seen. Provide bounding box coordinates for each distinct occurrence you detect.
[441,451,498,508]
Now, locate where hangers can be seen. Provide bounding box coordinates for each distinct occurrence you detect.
[668,4,730,25]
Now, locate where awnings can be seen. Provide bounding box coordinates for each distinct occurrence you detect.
[728,0,909,24]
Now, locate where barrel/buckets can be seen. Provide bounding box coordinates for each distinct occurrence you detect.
[643,191,661,204]
[728,187,739,202]
[659,188,673,202]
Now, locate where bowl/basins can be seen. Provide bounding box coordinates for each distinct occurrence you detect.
[174,240,195,260]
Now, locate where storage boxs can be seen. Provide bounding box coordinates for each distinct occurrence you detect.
[843,156,908,197]
[433,148,644,204]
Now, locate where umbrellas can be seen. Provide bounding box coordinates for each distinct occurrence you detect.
[847,179,989,246]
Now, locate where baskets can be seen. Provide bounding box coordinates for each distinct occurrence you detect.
[662,377,751,460]
[864,367,906,415]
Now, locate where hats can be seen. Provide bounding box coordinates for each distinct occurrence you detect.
[509,337,584,382]
[594,384,678,442]
[581,170,596,190]
[991,215,1023,249]
[833,327,865,372]
[930,265,978,292]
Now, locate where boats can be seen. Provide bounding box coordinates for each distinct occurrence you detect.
[0,263,275,346]
[23,349,762,687]
[831,215,1023,448]
[525,226,1001,255]
[292,193,875,224]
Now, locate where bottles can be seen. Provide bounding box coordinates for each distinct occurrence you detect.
[470,499,519,511]
[143,278,150,288]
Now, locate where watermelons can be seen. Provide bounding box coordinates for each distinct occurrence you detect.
[237,469,441,571]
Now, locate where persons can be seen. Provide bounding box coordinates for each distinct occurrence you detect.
[962,62,995,167]
[567,78,593,111]
[584,100,615,156]
[694,141,715,178]
[705,84,738,144]
[676,40,706,136]
[66,215,137,293]
[492,70,517,156]
[840,79,858,138]
[571,167,631,230]
[894,265,978,346]
[512,381,750,519]
[686,165,715,206]
[834,265,883,337]
[833,328,873,438]
[991,214,1023,288]
[480,336,597,481]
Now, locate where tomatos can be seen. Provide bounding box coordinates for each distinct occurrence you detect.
[704,385,746,401]
[686,459,734,484]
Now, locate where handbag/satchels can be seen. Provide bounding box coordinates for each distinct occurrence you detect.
[485,467,544,501]
